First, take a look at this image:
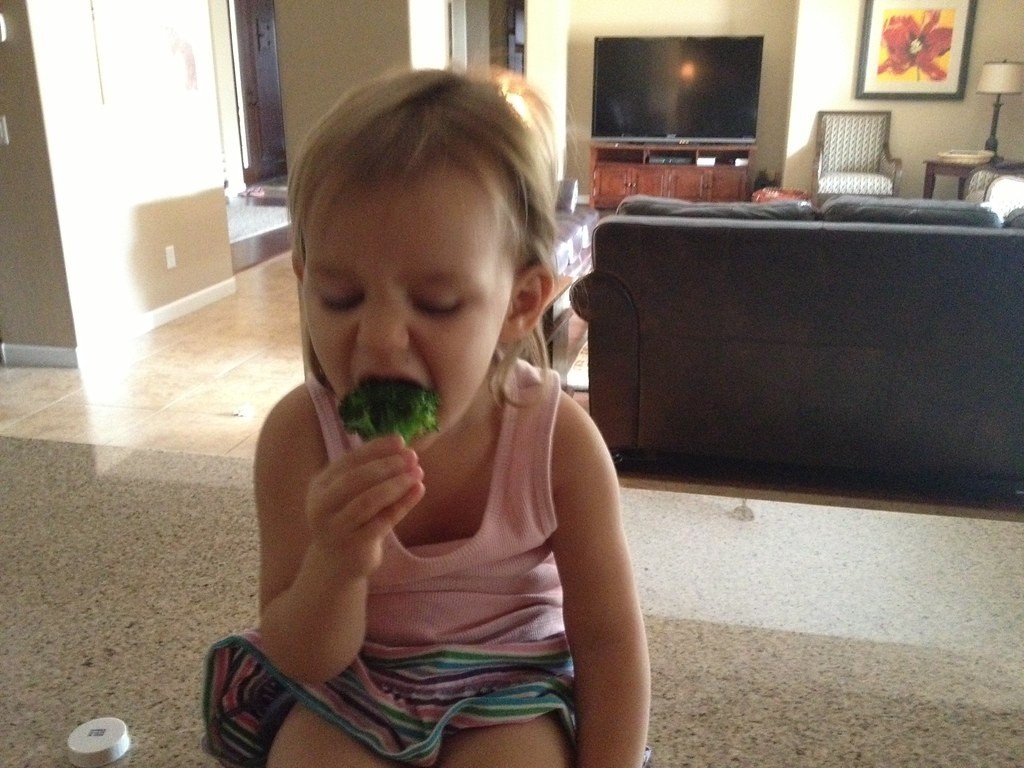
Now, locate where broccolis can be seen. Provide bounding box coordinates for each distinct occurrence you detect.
[336,377,442,447]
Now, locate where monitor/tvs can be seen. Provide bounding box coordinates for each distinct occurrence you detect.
[592,35,764,145]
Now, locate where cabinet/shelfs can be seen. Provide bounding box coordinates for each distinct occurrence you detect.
[587,143,758,211]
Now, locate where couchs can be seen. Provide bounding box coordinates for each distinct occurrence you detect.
[569,215,1024,500]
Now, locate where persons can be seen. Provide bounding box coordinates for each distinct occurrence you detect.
[253,64,655,767]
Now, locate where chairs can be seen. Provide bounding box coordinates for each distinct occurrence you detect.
[810,111,903,202]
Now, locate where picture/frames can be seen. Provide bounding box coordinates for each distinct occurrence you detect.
[855,0,980,102]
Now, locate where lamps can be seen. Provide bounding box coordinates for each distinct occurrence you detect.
[974,60,1024,161]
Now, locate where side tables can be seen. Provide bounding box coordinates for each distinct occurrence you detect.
[923,160,1024,199]
